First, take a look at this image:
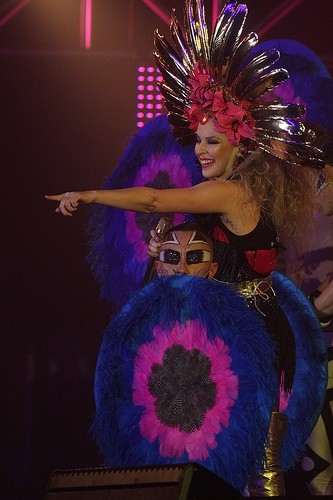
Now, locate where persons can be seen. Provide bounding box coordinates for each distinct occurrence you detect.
[44,78,297,500]
[147,223,218,283]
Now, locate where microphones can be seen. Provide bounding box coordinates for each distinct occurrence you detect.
[141,217,173,289]
